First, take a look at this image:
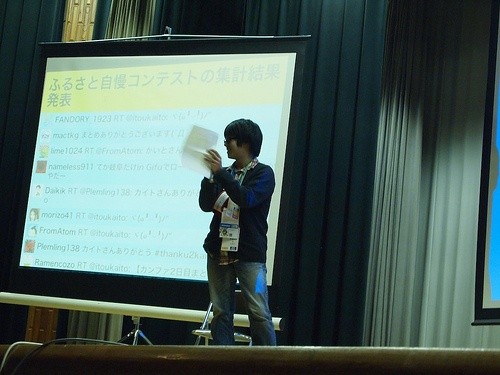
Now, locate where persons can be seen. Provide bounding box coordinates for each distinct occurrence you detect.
[198,120,277,347]
